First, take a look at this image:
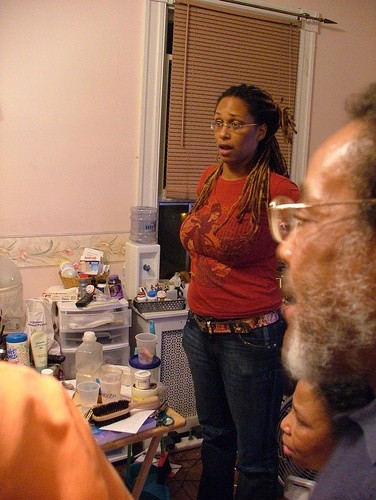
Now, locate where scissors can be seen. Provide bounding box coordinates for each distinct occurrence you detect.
[154,406,174,427]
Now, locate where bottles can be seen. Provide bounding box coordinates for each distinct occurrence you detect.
[76,331,103,393]
[108,274,123,299]
[80,279,87,298]
[97,279,107,294]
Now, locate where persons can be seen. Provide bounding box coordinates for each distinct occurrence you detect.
[179,83,302,500]
[267,82,376,500]
[279,377,336,471]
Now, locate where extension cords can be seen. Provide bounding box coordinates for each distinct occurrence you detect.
[144,435,204,456]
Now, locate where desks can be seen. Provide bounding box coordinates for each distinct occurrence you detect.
[63,381,187,499]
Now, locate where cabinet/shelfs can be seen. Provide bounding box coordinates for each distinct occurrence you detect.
[51,287,133,380]
[129,298,199,455]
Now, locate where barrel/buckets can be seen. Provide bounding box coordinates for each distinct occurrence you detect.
[130,206,158,243]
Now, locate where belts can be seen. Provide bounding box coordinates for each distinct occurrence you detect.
[193,312,280,334]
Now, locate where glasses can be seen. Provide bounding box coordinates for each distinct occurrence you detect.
[210,118,259,131]
[267,193,375,244]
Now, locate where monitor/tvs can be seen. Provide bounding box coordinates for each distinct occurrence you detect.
[157,202,195,279]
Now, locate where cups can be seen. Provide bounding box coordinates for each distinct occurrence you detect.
[76,381,100,414]
[100,367,123,382]
[100,374,122,405]
[135,333,158,365]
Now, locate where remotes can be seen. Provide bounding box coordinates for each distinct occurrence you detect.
[48,355,65,363]
[75,294,93,307]
